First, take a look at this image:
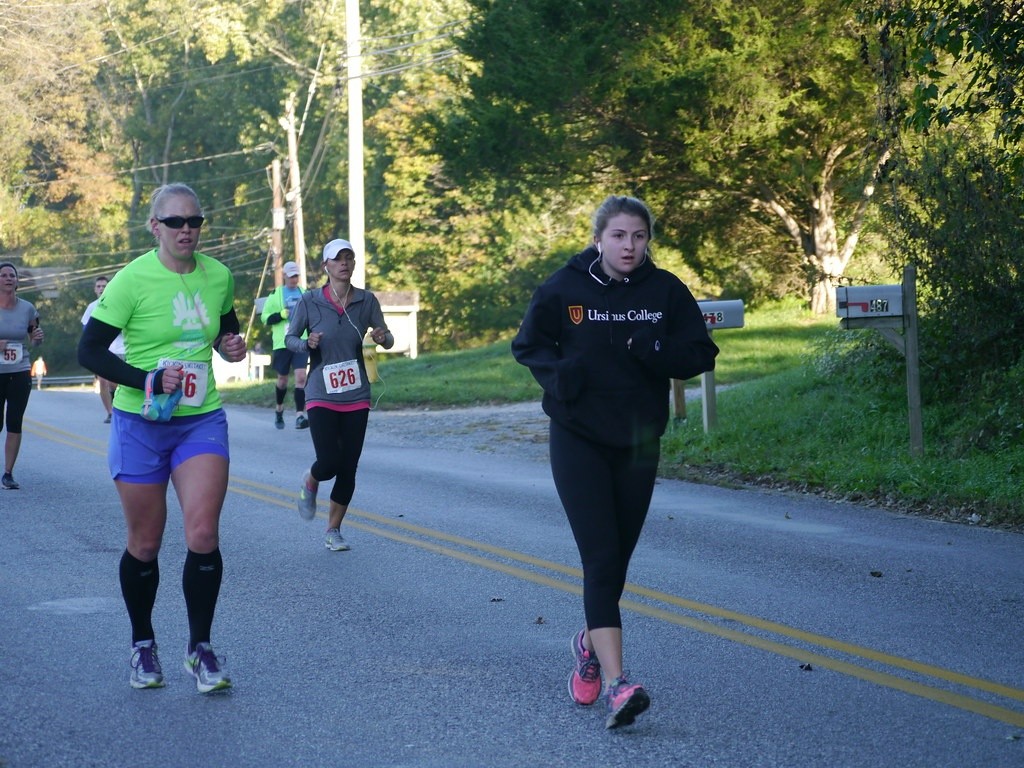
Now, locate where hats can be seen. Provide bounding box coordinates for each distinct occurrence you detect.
[283,262,300,277]
[323,238,355,262]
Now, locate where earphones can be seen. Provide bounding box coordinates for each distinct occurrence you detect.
[155,228,161,236]
[324,265,328,273]
[645,247,647,254]
[597,242,602,253]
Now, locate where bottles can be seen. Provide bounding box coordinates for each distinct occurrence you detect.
[141,393,170,421]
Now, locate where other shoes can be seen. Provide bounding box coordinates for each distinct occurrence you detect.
[105,413,111,422]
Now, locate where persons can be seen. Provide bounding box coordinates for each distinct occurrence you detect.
[511,198,719,730]
[259,260,314,429]
[77,181,248,694]
[284,238,394,551]
[0,263,44,490]
[80,277,127,423]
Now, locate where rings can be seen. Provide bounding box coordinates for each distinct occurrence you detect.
[378,335,381,340]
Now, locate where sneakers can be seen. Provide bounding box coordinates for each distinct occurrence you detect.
[2,474,19,489]
[275,411,286,429]
[606,669,650,728]
[184,640,231,692]
[298,472,319,520]
[295,415,309,429]
[130,639,164,688]
[324,527,349,550]
[567,629,601,704]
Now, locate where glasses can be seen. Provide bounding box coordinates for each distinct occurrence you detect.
[155,215,205,229]
